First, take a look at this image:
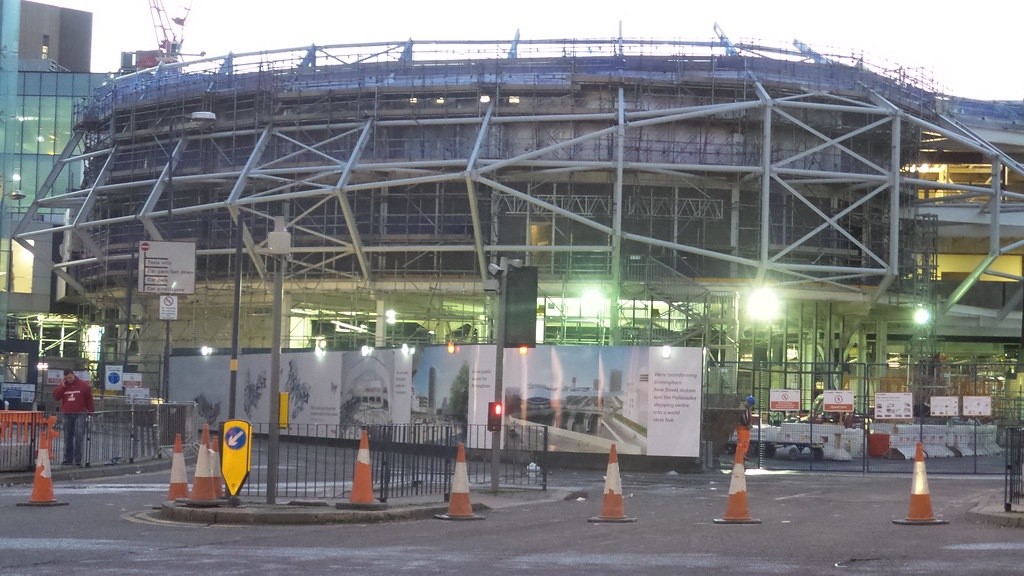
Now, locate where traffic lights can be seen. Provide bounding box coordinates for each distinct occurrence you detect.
[487,402,502,433]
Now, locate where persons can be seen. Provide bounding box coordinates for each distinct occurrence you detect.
[54,368,95,467]
[739,397,755,429]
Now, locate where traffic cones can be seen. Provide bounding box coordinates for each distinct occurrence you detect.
[336,427,387,510]
[209,436,226,499]
[434,442,487,521]
[587,443,638,523]
[16,422,70,507]
[891,441,949,526]
[185,423,220,507]
[711,445,761,525]
[151,432,190,509]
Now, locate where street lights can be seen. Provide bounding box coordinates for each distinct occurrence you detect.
[0,190,26,220]
[160,112,216,459]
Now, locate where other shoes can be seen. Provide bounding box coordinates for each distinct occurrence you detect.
[62,459,74,466]
[76,462,84,467]
